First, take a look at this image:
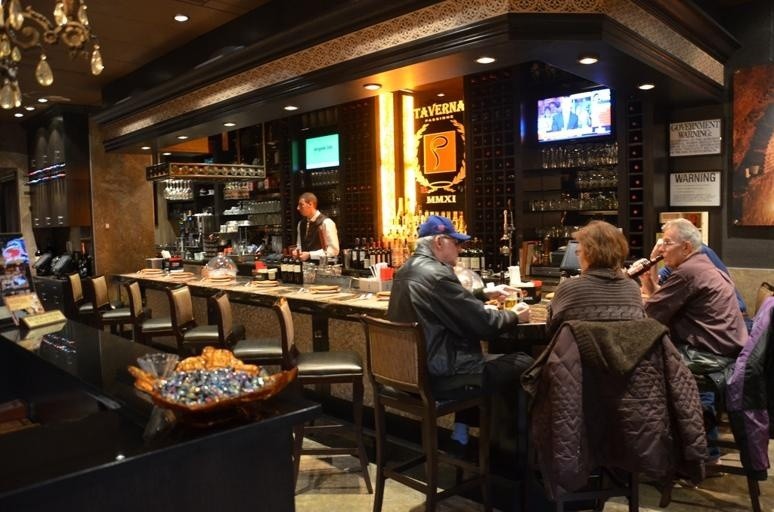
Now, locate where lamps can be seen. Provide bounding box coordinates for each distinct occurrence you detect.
[0,0,106,106]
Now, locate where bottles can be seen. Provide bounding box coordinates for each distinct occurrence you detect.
[574,170,619,188]
[528,191,618,210]
[281,247,287,282]
[534,223,579,266]
[288,248,293,283]
[541,141,619,168]
[222,179,253,199]
[452,210,487,277]
[627,255,663,279]
[236,200,282,224]
[387,198,451,267]
[352,236,393,269]
[293,248,304,284]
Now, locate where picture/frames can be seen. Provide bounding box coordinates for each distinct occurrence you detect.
[667,170,722,209]
[658,209,709,248]
[725,52,773,241]
[664,119,722,157]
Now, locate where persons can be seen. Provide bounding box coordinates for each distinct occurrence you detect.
[536,93,601,131]
[291,192,340,265]
[649,229,754,335]
[545,219,648,345]
[630,218,748,477]
[385,215,537,457]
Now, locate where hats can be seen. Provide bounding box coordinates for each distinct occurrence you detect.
[417,215,472,241]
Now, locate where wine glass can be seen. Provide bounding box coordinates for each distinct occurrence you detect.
[163,178,194,201]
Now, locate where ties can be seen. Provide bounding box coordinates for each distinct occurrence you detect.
[306,220,311,237]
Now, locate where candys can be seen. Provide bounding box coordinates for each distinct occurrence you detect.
[155,368,265,404]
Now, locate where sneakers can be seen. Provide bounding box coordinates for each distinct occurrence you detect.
[442,438,479,455]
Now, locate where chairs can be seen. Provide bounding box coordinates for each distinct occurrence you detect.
[752,281,773,317]
[526,321,642,512]
[360,314,494,511]
[656,295,774,511]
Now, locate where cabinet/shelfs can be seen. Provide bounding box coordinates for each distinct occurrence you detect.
[294,165,342,249]
[32,276,89,318]
[466,68,516,259]
[22,103,91,228]
[280,139,300,248]
[158,179,217,256]
[217,170,284,254]
[338,94,378,249]
[627,96,644,261]
[514,141,619,248]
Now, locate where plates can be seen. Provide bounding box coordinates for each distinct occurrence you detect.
[126,345,299,414]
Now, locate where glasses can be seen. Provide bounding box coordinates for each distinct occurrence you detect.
[575,247,582,254]
[440,236,462,246]
[663,240,678,247]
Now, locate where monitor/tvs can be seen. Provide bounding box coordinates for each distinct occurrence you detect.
[304,132,341,170]
[533,87,617,145]
